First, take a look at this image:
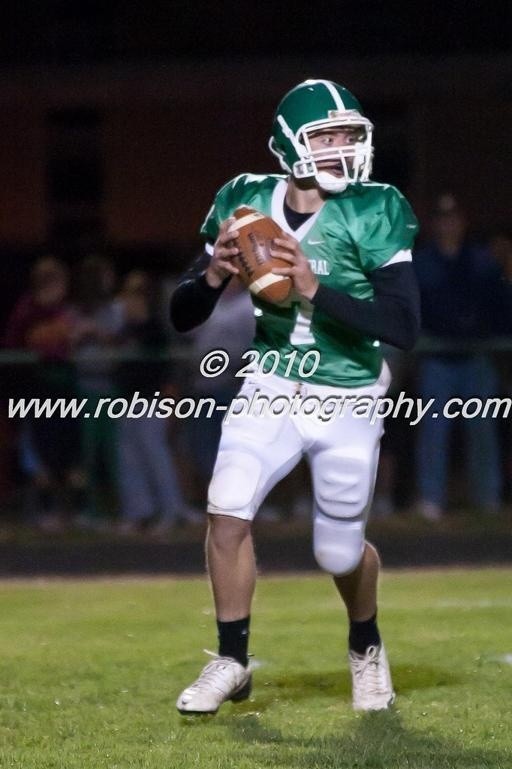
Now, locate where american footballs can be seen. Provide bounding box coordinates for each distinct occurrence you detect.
[227,204,292,305]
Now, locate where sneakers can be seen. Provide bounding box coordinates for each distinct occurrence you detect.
[174,650,253,716]
[346,635,394,717]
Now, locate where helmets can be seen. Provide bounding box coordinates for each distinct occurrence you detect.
[267,79,375,179]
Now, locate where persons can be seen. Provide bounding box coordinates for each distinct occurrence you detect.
[172,78,422,719]
[2,180,512,539]
